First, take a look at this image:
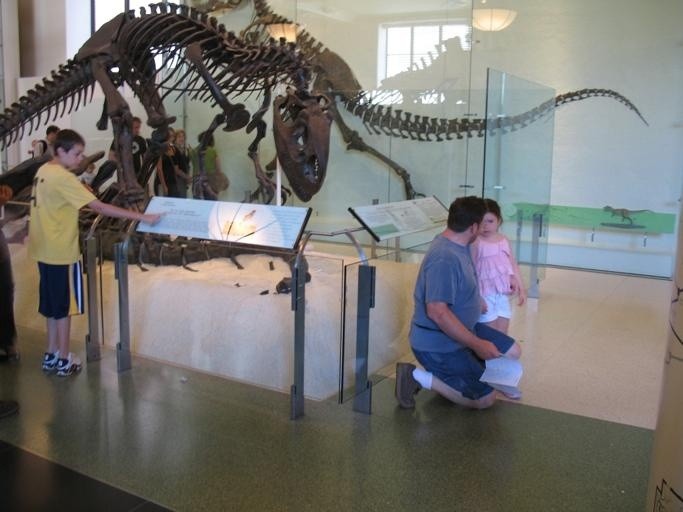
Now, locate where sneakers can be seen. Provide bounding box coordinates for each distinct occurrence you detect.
[42,349,60,372]
[503,387,522,399]
[56,353,83,377]
[395,362,422,409]
[0,347,20,362]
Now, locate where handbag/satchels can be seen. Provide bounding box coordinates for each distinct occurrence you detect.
[211,173,229,192]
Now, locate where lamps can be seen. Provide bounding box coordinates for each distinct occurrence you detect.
[471,9,519,33]
[267,21,300,42]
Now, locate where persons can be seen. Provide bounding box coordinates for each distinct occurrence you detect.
[469,199,526,399]
[27,129,161,376]
[31,118,220,201]
[0,186,20,364]
[395,196,522,409]
